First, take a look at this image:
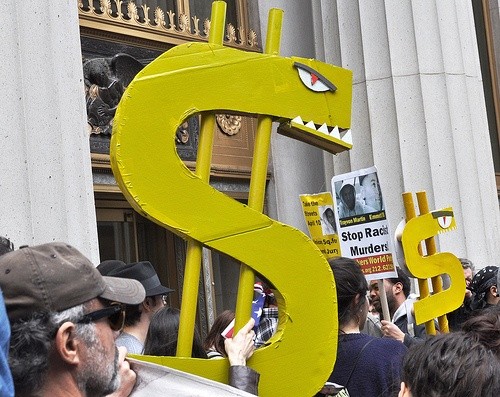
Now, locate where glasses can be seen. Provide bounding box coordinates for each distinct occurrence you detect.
[47,301,125,339]
[156,294,168,301]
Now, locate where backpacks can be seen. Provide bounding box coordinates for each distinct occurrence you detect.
[314,337,379,397]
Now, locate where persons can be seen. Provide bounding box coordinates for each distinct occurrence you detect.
[0,236,279,397]
[323,256,409,397]
[360,257,500,347]
[337,172,384,218]
[321,207,338,235]
[392,329,500,397]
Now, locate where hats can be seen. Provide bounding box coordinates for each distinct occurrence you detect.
[0,240,146,322]
[107,261,176,296]
[95,262,131,277]
